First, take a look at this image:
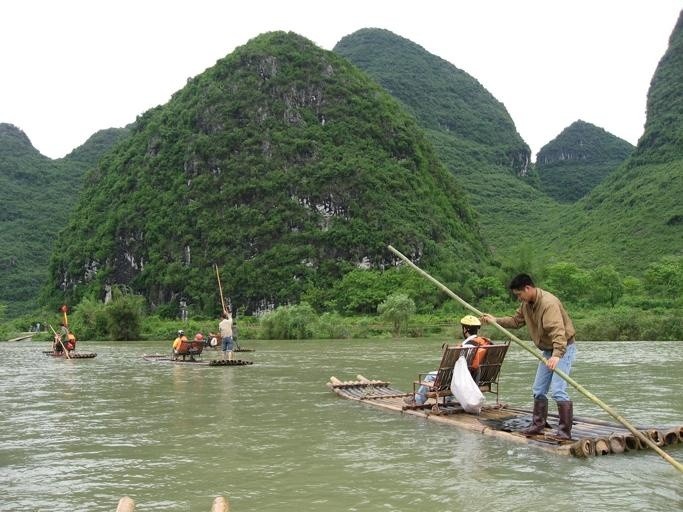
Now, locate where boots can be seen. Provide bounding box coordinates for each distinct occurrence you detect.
[520,399,549,437]
[545,400,574,443]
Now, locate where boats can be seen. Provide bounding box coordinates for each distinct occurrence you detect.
[115,495,228,512]
[200,334,255,353]
[42,349,97,359]
[326,340,682,460]
[142,341,252,366]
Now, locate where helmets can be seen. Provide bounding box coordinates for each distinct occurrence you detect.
[459,314,482,328]
[177,329,184,335]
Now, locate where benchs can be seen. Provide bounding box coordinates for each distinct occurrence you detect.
[412,340,515,414]
[171,340,206,361]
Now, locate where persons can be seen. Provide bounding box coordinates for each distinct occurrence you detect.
[403,314,491,405]
[53,323,76,354]
[478,272,576,441]
[171,310,241,362]
